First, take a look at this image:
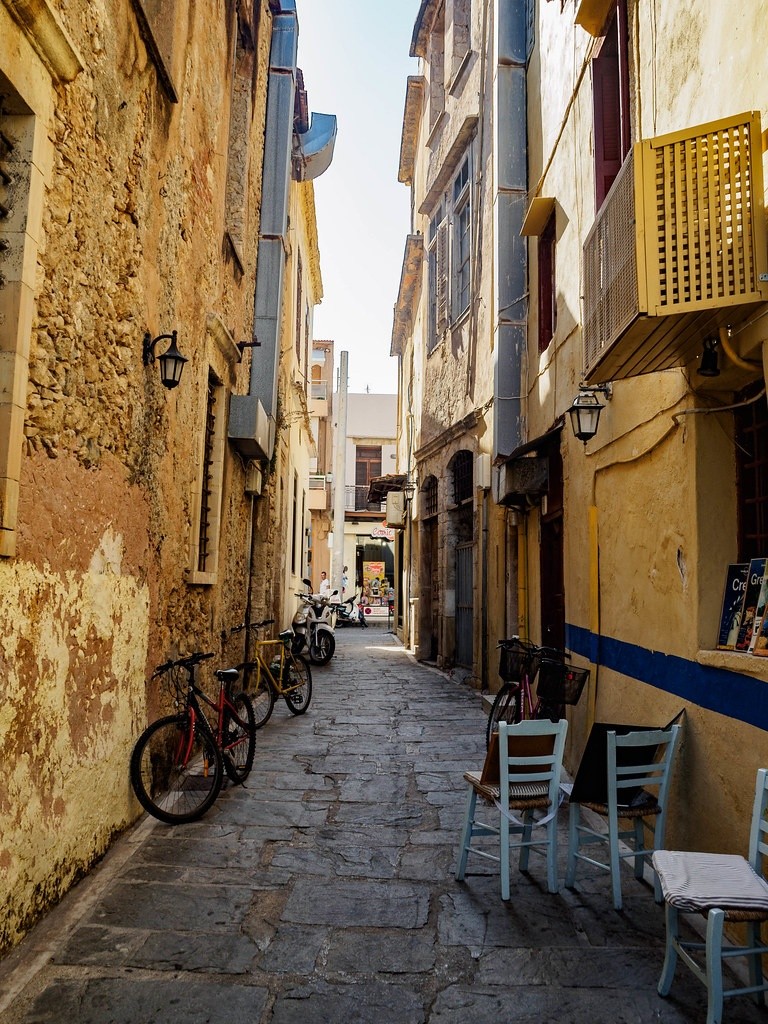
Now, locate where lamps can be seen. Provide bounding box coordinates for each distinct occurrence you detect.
[564,382,612,446]
[403,480,419,502]
[142,330,189,391]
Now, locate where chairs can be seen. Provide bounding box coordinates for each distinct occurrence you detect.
[558,708,684,909]
[454,717,569,900]
[651,768,768,1024]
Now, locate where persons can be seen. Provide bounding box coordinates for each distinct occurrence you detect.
[319,571,330,595]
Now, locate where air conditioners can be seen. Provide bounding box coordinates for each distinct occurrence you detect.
[385,491,406,527]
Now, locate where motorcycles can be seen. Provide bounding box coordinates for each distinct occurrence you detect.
[289,578,339,666]
[331,592,369,628]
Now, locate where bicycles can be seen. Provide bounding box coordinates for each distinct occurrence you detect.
[486,633,590,755]
[228,618,313,728]
[127,650,258,825]
